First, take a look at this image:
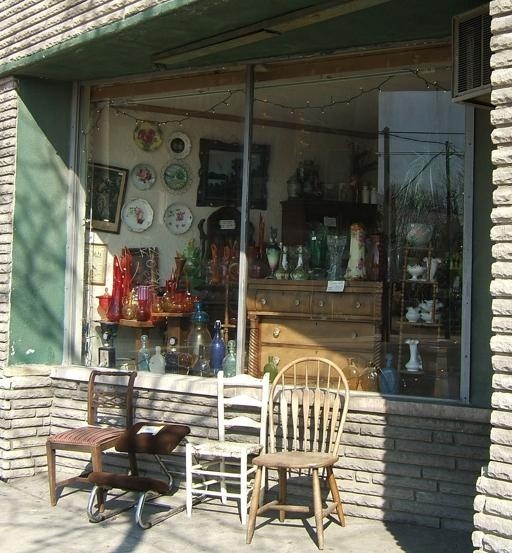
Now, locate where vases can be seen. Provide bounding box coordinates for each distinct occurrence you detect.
[404,256,444,324]
[404,338,423,371]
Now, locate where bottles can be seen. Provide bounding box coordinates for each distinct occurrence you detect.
[183,213,381,282]
[263,354,279,384]
[137,310,237,378]
[340,353,400,394]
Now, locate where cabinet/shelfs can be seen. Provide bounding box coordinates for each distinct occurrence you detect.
[245,279,384,392]
[279,199,378,250]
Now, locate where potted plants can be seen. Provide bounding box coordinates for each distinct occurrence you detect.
[392,141,462,248]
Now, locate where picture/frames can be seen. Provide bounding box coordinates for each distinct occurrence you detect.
[85,162,129,235]
[195,136,271,210]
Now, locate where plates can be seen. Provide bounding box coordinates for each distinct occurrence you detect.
[164,164,188,191]
[164,202,194,234]
[166,132,192,159]
[133,120,162,151]
[121,198,155,234]
[131,163,156,190]
